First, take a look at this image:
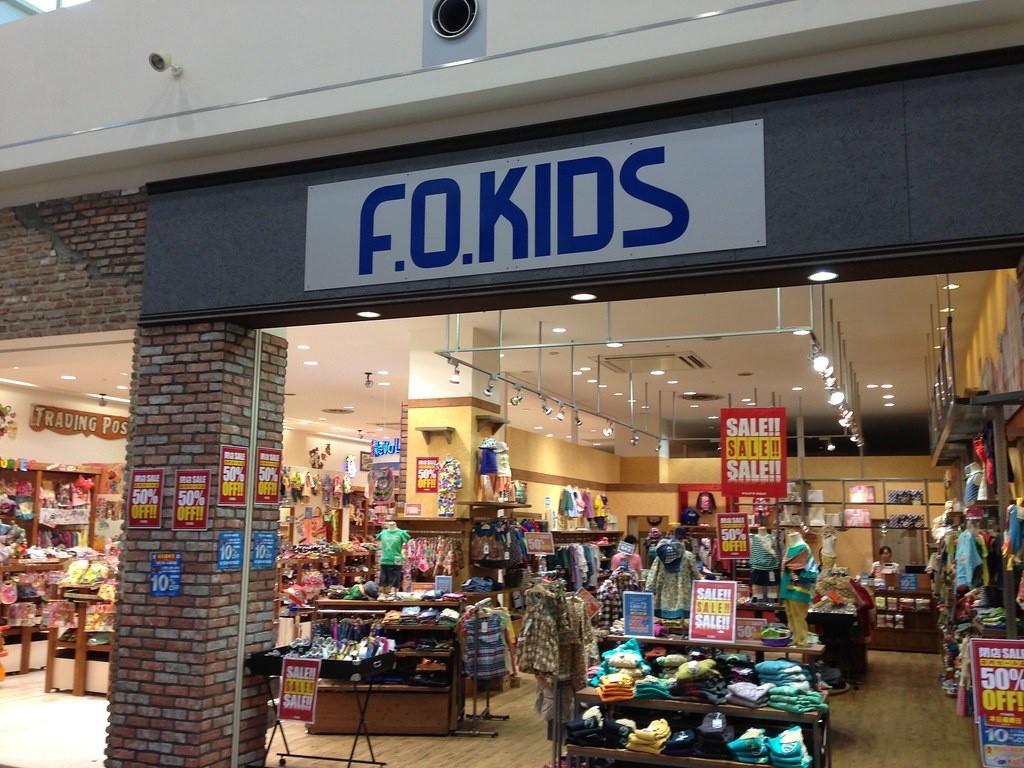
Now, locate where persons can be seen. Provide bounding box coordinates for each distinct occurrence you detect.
[750,526,781,602]
[561,485,607,529]
[779,532,816,646]
[821,525,838,573]
[376,521,410,593]
[480,437,513,504]
[434,453,462,516]
[871,546,901,579]
[964,465,983,501]
[611,536,643,580]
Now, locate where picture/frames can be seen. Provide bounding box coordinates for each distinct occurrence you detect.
[360,451,372,471]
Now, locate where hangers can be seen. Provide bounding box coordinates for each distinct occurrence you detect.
[523,569,577,598]
[403,529,465,543]
[457,597,508,623]
[471,517,539,532]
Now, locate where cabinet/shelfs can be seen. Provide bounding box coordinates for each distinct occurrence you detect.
[349,486,399,542]
[331,551,378,589]
[0,458,40,551]
[45,584,116,696]
[279,557,336,638]
[0,559,68,674]
[393,425,470,521]
[565,633,832,768]
[550,530,624,594]
[306,594,467,735]
[471,415,533,508]
[966,500,1024,637]
[35,463,102,551]
[731,479,948,528]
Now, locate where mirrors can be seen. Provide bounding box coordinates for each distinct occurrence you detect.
[279,506,295,546]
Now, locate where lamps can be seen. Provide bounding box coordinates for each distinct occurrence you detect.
[448,358,663,452]
[881,525,888,534]
[806,338,865,449]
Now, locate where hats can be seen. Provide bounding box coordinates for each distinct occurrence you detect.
[283,583,307,606]
[493,441,509,453]
[478,438,497,449]
[657,539,685,572]
